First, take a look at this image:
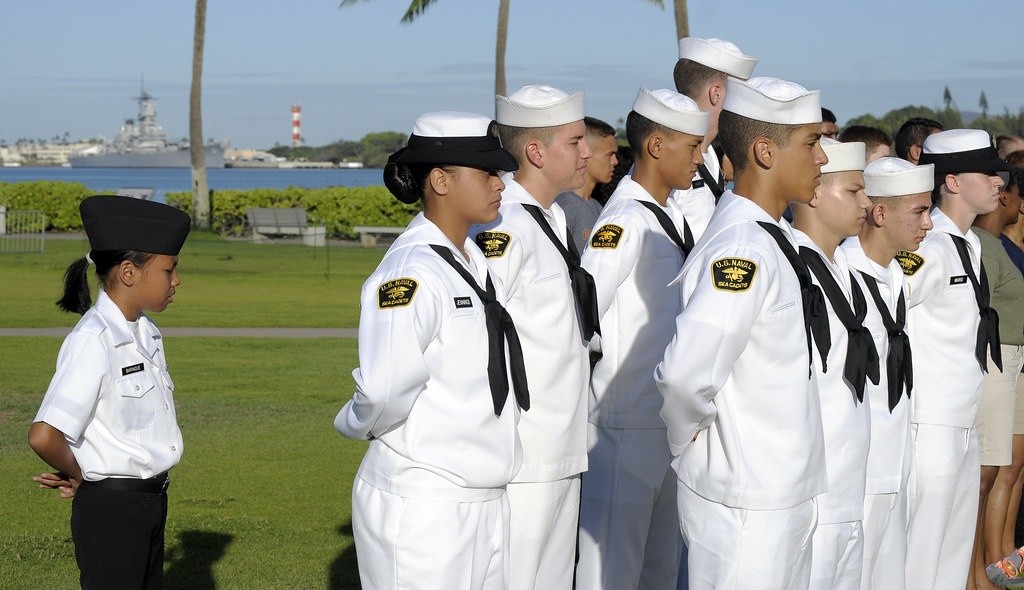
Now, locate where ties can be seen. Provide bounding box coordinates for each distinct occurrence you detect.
[861,272,911,414]
[636,199,694,262]
[431,244,530,417]
[950,233,1003,373]
[696,164,724,207]
[799,245,880,403]
[756,221,831,380]
[522,204,604,341]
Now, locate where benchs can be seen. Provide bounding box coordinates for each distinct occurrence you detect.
[246,208,325,247]
[354,226,407,248]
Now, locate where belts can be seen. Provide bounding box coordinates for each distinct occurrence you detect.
[98,474,170,495]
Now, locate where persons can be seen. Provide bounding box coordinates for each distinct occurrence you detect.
[332,113,531,590]
[581,32,1024,590]
[552,110,620,258]
[466,85,605,590]
[27,195,191,590]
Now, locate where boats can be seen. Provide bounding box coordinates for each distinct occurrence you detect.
[67,74,225,169]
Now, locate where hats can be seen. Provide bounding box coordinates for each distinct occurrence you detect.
[80,195,191,255]
[818,138,866,173]
[496,85,584,128]
[723,75,823,124]
[862,157,935,196]
[918,129,1014,173]
[678,37,757,79]
[632,87,708,137]
[401,111,519,172]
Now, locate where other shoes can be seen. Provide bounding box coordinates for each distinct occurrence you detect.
[1001,548,1024,571]
[986,560,1024,590]
